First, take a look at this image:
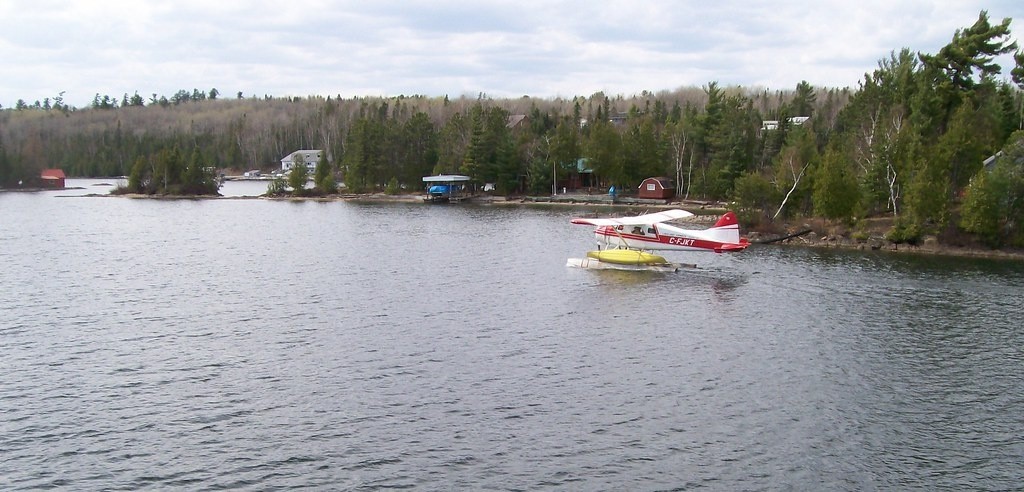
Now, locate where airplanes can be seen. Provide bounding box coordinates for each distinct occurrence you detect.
[570,209,753,272]
